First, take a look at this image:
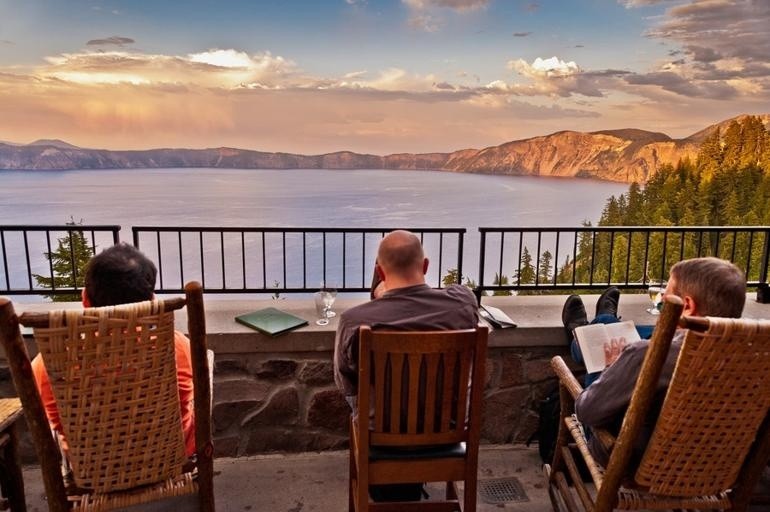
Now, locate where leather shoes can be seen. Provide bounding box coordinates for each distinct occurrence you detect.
[561,292,588,345]
[595,288,621,319]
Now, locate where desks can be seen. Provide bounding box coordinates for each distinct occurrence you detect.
[1,396,26,509]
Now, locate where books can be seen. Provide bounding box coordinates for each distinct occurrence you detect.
[234,307,309,339]
[571,318,642,375]
[478,303,516,331]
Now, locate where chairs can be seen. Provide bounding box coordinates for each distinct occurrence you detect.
[543,293,770,512]
[1,281,222,512]
[348,325,489,512]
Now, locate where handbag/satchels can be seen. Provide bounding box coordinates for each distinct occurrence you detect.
[472,286,518,331]
[368,483,431,503]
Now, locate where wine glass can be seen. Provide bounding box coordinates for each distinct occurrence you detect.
[645,278,666,315]
[314,283,337,326]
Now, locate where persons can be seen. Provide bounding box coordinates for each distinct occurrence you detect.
[332,229,482,454]
[29,239,196,485]
[562,255,746,480]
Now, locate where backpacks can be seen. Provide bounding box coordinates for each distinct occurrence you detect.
[525,373,596,477]
